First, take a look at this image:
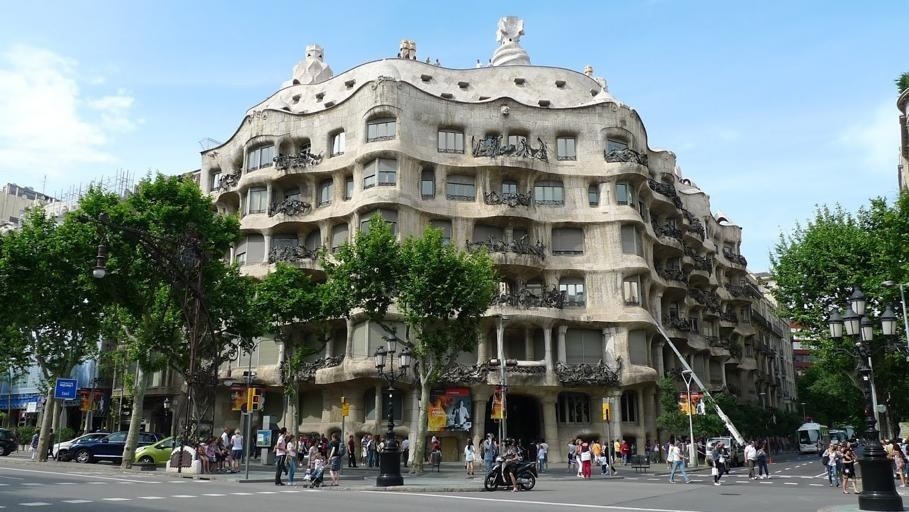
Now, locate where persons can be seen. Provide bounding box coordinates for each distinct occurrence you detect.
[464,437,475,475]
[452,400,470,425]
[360,432,410,470]
[712,441,731,486]
[426,57,432,65]
[30,428,40,461]
[293,79,300,86]
[568,439,636,479]
[645,440,706,483]
[479,433,549,492]
[822,440,909,494]
[743,439,772,480]
[413,55,417,60]
[195,427,243,473]
[476,59,482,69]
[487,59,491,67]
[404,53,409,58]
[47,428,56,463]
[272,427,358,489]
[433,59,441,66]
[429,434,442,473]
[397,52,402,58]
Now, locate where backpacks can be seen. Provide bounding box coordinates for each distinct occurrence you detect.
[337,442,346,456]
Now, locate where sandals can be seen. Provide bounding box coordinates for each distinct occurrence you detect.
[854,491,861,494]
[843,491,848,494]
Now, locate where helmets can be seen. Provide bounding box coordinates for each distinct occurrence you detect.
[502,437,513,447]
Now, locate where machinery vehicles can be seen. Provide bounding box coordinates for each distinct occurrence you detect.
[651,319,756,468]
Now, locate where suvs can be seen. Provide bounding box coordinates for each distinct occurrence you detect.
[0,428,18,457]
[69,429,166,466]
[51,432,113,462]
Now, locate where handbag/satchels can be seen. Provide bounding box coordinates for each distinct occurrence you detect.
[711,467,719,476]
[305,468,311,473]
[822,457,830,465]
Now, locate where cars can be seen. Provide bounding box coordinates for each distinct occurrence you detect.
[134,435,205,467]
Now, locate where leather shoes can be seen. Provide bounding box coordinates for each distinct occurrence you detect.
[835,482,840,487]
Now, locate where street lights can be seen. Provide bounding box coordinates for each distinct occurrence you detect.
[479,357,523,457]
[801,401,808,422]
[824,283,902,512]
[681,369,701,469]
[75,212,205,476]
[760,389,769,433]
[372,326,413,484]
[219,330,287,480]
[880,276,908,344]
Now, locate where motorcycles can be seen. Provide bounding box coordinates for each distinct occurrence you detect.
[484,454,538,491]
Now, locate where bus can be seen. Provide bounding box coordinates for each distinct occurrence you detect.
[841,425,857,446]
[828,429,848,446]
[796,423,830,454]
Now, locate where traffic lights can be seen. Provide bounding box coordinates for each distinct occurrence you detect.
[247,388,263,414]
